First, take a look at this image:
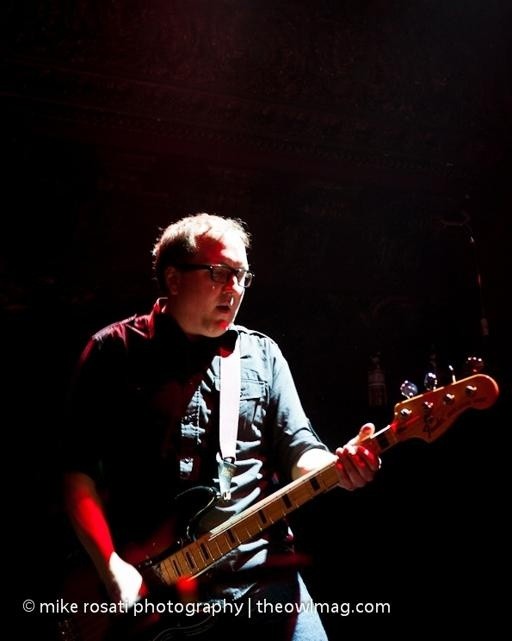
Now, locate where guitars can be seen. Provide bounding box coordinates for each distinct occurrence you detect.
[57,356,499,641]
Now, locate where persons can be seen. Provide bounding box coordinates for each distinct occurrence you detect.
[56,212,381,641]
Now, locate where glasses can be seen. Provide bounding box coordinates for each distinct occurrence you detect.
[173,261,257,286]
